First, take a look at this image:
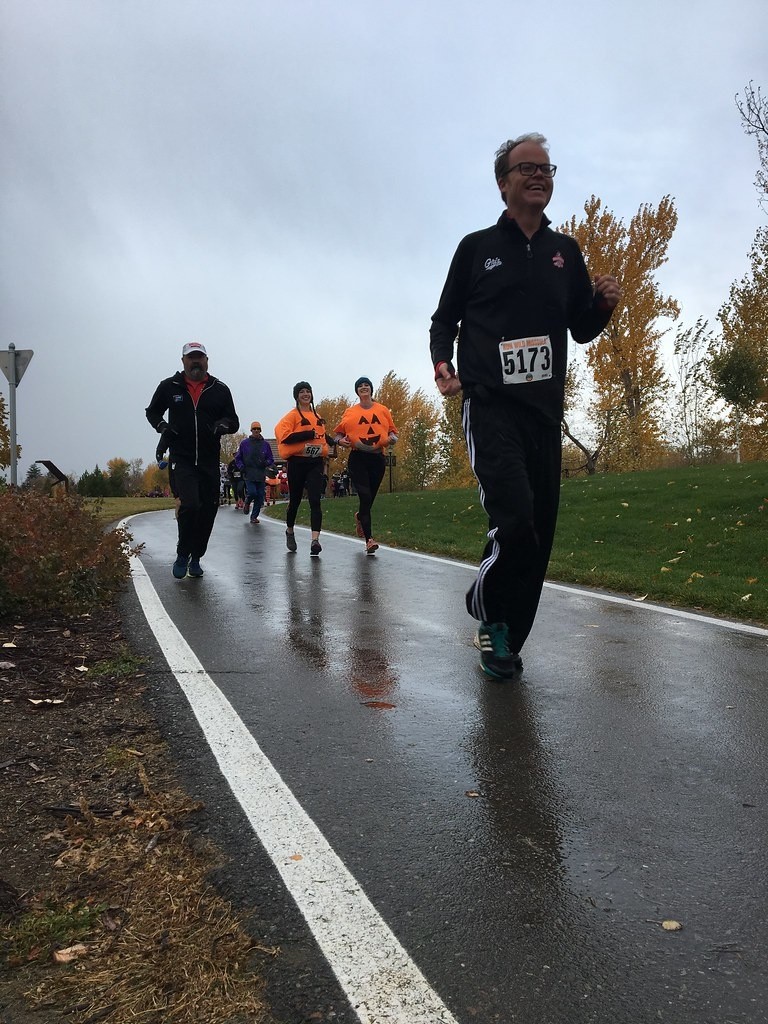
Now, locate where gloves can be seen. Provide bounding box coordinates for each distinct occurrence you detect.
[214,420,229,436]
[158,460,168,469]
[240,467,246,477]
[161,428,185,442]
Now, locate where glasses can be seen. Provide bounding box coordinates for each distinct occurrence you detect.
[505,162,557,178]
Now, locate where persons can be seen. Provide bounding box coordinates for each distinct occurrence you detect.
[319,467,351,500]
[276,382,334,555]
[336,377,398,553]
[433,133,620,672]
[146,341,288,579]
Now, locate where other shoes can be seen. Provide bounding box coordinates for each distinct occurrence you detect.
[235,506,239,509]
[267,503,270,507]
[220,498,231,505]
[238,501,243,508]
[320,496,324,500]
[273,502,275,505]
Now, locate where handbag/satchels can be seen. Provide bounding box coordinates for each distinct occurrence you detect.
[264,465,277,478]
[339,485,344,489]
[337,480,343,484]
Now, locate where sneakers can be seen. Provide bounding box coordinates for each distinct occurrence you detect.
[188,559,204,577]
[472,621,513,660]
[365,540,378,556]
[251,518,259,523]
[244,504,249,514]
[354,512,365,538]
[286,529,297,552]
[173,549,191,580]
[309,540,321,556]
[480,654,523,679]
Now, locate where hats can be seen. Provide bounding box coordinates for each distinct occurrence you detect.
[251,422,261,429]
[293,381,326,426]
[283,464,287,467]
[182,342,208,357]
[355,377,373,394]
